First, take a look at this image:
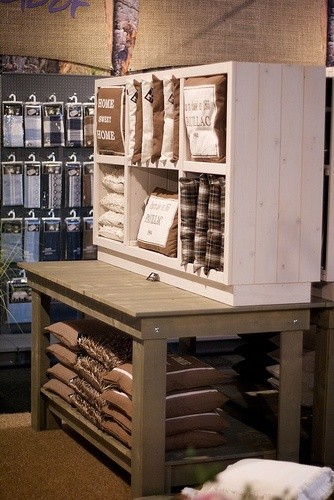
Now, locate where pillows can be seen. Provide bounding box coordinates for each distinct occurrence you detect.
[39,317,316,455]
[93,74,225,274]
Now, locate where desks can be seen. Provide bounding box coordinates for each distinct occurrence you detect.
[19,256,333,497]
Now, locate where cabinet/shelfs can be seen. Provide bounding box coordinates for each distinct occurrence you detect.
[94,66,328,305]
